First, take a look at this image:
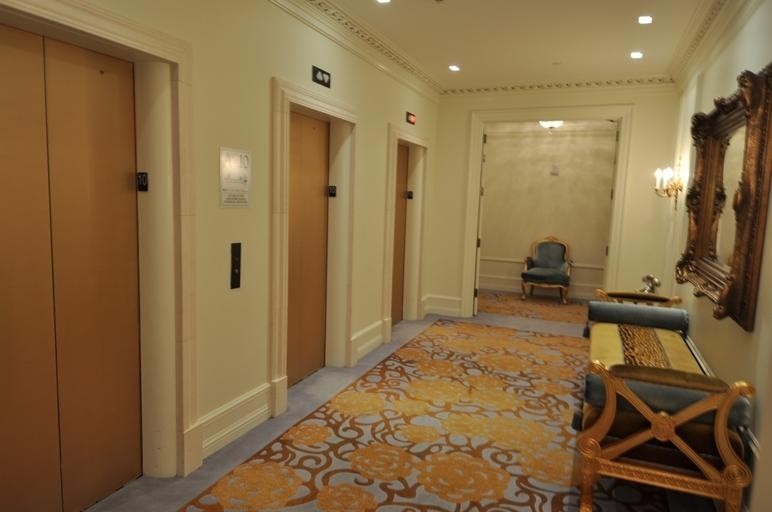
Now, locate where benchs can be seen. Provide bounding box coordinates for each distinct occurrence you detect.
[575,285,756,512]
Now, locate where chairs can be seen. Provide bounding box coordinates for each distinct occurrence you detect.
[520,235,576,305]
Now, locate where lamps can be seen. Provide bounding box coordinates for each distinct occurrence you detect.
[651,165,683,212]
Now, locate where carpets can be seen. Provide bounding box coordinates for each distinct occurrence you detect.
[477,290,592,326]
[176,318,720,512]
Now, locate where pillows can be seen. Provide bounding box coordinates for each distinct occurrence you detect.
[587,299,689,332]
[575,372,752,429]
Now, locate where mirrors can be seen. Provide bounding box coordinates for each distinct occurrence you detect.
[673,59,771,335]
